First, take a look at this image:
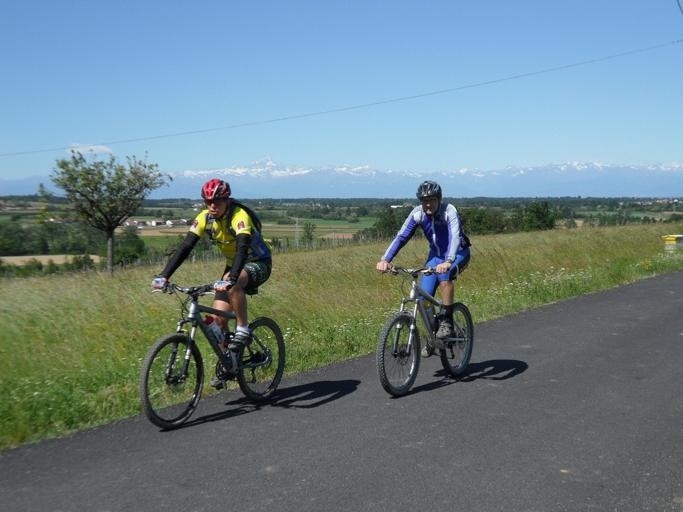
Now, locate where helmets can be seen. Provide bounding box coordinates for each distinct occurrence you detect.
[416,181,442,204]
[201,178,231,200]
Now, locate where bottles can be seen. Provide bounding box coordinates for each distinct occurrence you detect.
[423,300,434,322]
[204,315,226,344]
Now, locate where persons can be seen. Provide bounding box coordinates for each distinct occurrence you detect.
[151,178,272,388]
[375,180,471,358]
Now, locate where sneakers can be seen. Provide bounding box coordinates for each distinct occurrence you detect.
[421,344,435,359]
[229,329,253,349]
[209,367,235,386]
[436,321,454,338]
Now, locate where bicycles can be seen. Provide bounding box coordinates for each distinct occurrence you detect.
[139,275,287,430]
[374,261,474,398]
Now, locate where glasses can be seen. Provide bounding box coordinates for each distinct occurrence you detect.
[205,197,225,206]
